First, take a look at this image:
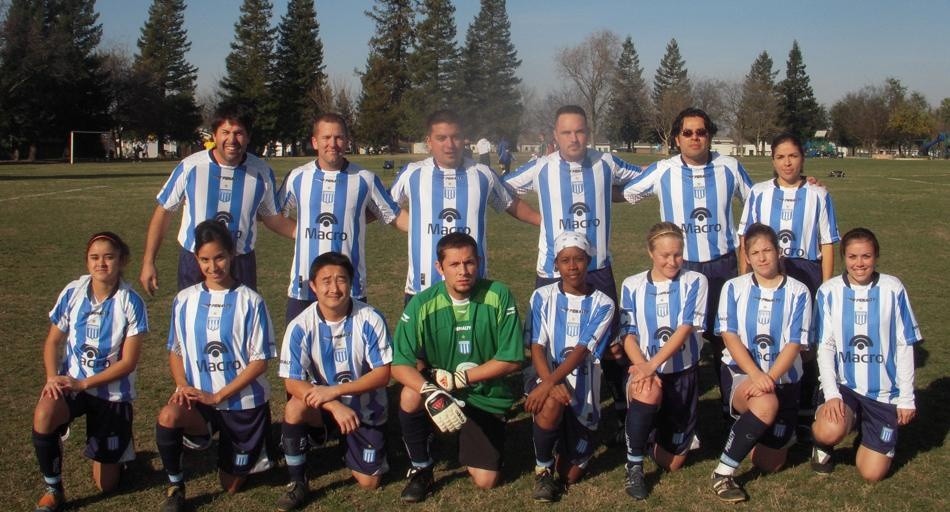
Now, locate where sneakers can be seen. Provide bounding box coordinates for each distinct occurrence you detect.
[277,476,310,512]
[625,462,650,500]
[400,462,436,502]
[708,468,746,502]
[158,481,186,512]
[810,439,837,476]
[533,464,560,503]
[34,485,65,512]
[794,407,816,432]
[602,411,627,451]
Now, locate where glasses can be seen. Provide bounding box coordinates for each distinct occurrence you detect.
[679,127,709,138]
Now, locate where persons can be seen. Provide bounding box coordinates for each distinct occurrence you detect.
[138,100,298,294]
[391,231,528,503]
[278,250,394,509]
[548,137,557,154]
[475,133,495,170]
[153,217,277,511]
[703,223,813,503]
[736,130,840,445]
[499,146,516,175]
[809,226,925,485]
[536,130,550,157]
[610,108,828,429]
[462,134,474,161]
[615,220,711,501]
[497,133,516,168]
[31,231,150,511]
[364,108,544,310]
[515,229,618,504]
[255,112,410,330]
[498,104,656,445]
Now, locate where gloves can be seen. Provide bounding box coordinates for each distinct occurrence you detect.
[420,380,468,434]
[419,367,471,392]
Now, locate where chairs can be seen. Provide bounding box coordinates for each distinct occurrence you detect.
[383,160,394,177]
